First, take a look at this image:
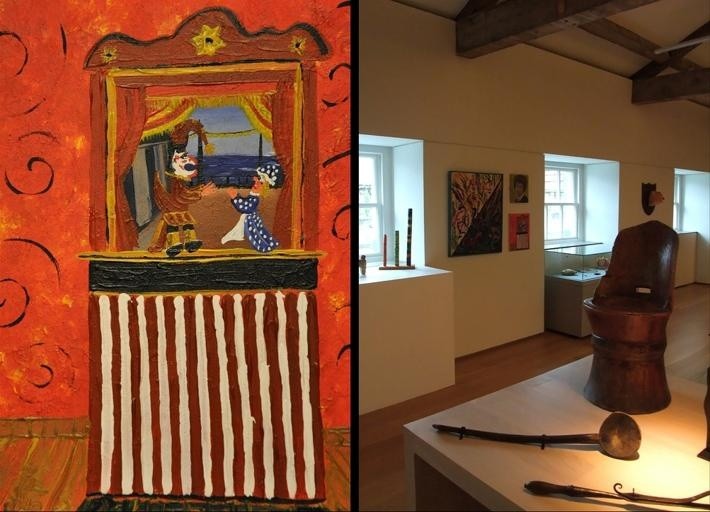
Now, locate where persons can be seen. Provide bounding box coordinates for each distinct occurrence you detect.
[512,175,528,203]
